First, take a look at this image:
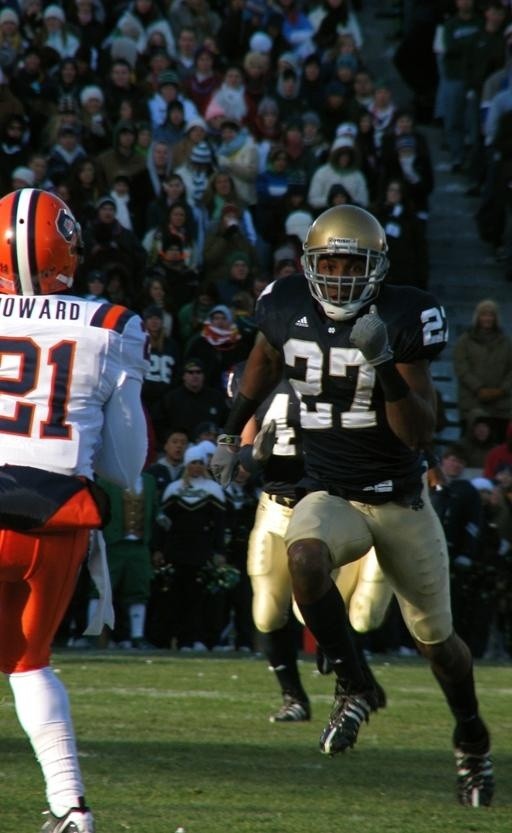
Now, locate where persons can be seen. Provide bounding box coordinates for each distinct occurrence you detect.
[240,371,394,722]
[211,202,494,806]
[0,187,148,830]
[0,0,512,659]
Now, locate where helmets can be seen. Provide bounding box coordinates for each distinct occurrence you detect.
[301,205,390,320]
[0,189,81,296]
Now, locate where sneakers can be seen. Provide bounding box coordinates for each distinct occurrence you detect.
[42,797,93,833]
[269,699,310,722]
[320,682,385,757]
[454,738,495,806]
